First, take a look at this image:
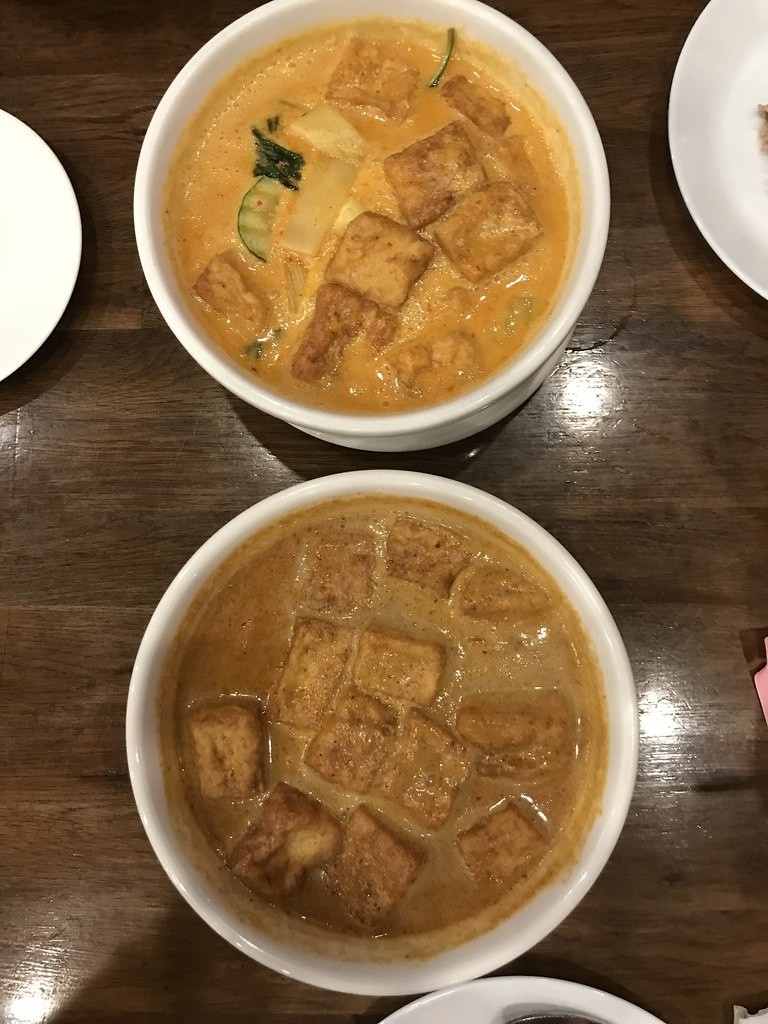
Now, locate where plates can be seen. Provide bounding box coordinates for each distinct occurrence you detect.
[0,106,82,380]
[668,0,768,302]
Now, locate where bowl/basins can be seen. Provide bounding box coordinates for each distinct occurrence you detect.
[125,470,640,997]
[132,0,612,451]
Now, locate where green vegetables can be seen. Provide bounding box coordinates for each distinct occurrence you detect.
[250,117,304,192]
[427,27,455,87]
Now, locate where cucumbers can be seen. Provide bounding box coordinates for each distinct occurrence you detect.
[237,178,279,262]
